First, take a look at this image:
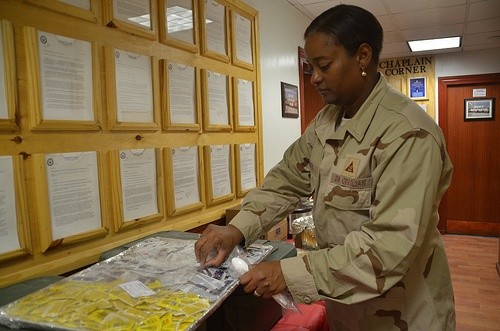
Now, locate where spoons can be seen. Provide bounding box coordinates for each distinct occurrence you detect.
[231,257,288,305]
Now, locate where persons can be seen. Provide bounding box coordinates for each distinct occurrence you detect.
[195,4,457,331]
[469,105,488,113]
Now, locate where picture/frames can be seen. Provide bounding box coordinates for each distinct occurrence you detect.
[280,80,299,119]
[464,97,495,122]
[408,76,426,99]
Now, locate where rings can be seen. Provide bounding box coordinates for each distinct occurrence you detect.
[254,291,260,296]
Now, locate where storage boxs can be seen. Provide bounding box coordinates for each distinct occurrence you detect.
[224,204,287,242]
